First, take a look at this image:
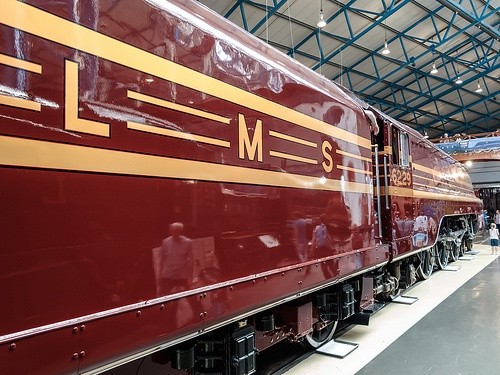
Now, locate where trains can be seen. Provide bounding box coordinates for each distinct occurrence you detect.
[0,0,485,375]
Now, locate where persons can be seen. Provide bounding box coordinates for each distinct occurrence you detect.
[162,208,483,331]
[483,210,489,232]
[489,223,499,255]
[494,210,500,235]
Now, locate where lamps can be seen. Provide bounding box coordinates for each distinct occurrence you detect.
[382,19,390,56]
[317,0,327,29]
[475,79,482,93]
[455,62,463,85]
[430,53,438,75]
